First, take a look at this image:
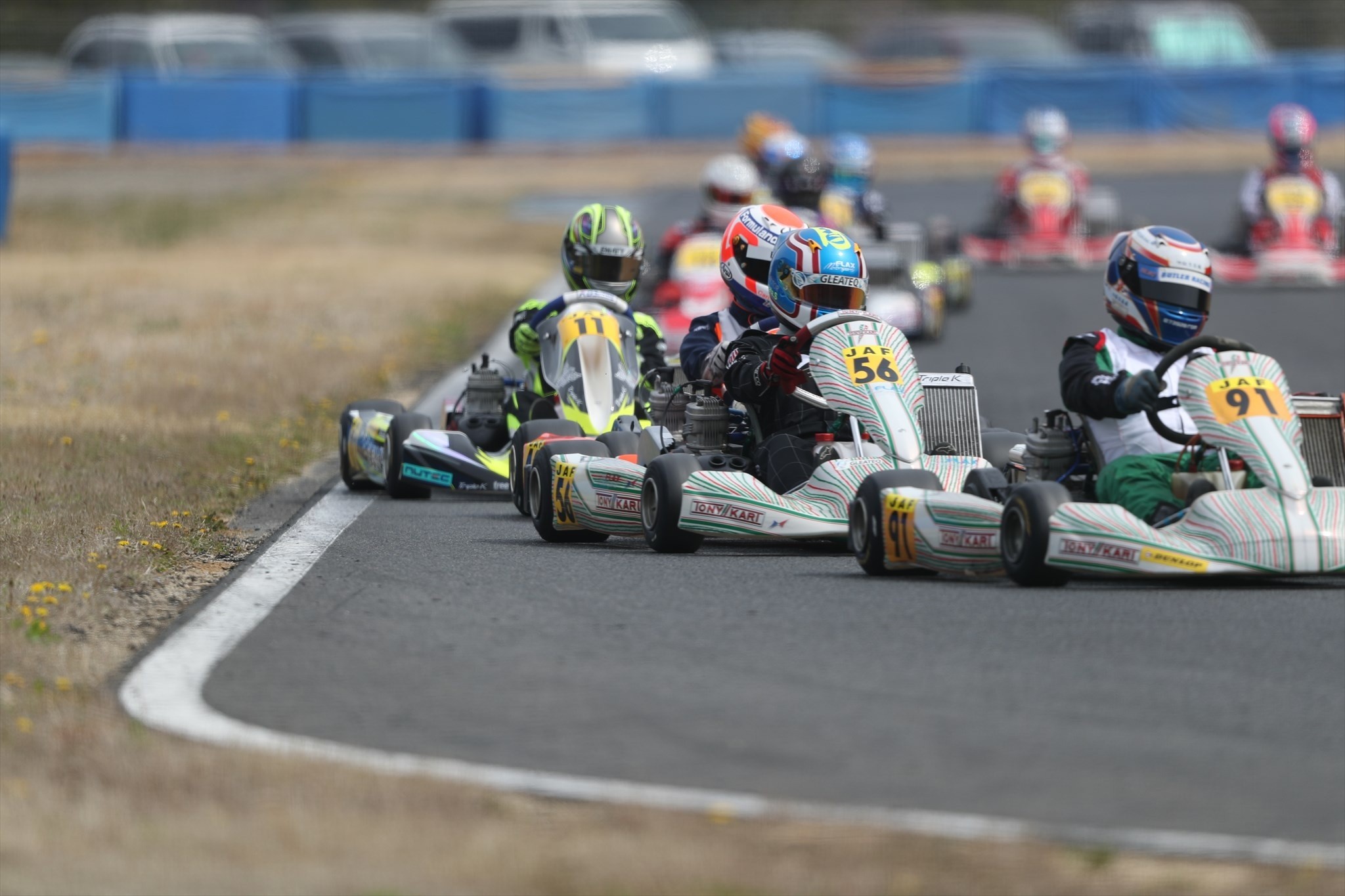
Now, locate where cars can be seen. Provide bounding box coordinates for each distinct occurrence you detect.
[270,11,475,87]
[52,8,296,75]
[713,9,1096,82]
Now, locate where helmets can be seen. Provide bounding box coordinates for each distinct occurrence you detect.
[827,131,875,175]
[701,154,759,218]
[563,202,646,303]
[1270,103,1316,159]
[720,205,809,316]
[767,227,870,333]
[1022,107,1070,155]
[1105,225,1213,348]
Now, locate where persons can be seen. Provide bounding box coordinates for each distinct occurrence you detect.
[655,110,887,394]
[992,105,1092,236]
[506,203,668,439]
[1240,102,1341,254]
[1058,225,1336,527]
[721,226,960,494]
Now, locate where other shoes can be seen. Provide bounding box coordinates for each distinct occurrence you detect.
[1184,478,1216,513]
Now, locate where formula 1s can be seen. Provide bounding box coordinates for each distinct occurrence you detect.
[847,334,1345,585]
[641,215,976,361]
[1189,172,1345,288]
[936,163,1128,276]
[330,288,1024,562]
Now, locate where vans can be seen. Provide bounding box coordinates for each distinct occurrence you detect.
[1063,0,1275,64]
[439,0,723,82]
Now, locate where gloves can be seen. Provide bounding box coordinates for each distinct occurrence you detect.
[1115,370,1166,412]
[708,341,730,377]
[514,323,541,356]
[764,336,802,379]
